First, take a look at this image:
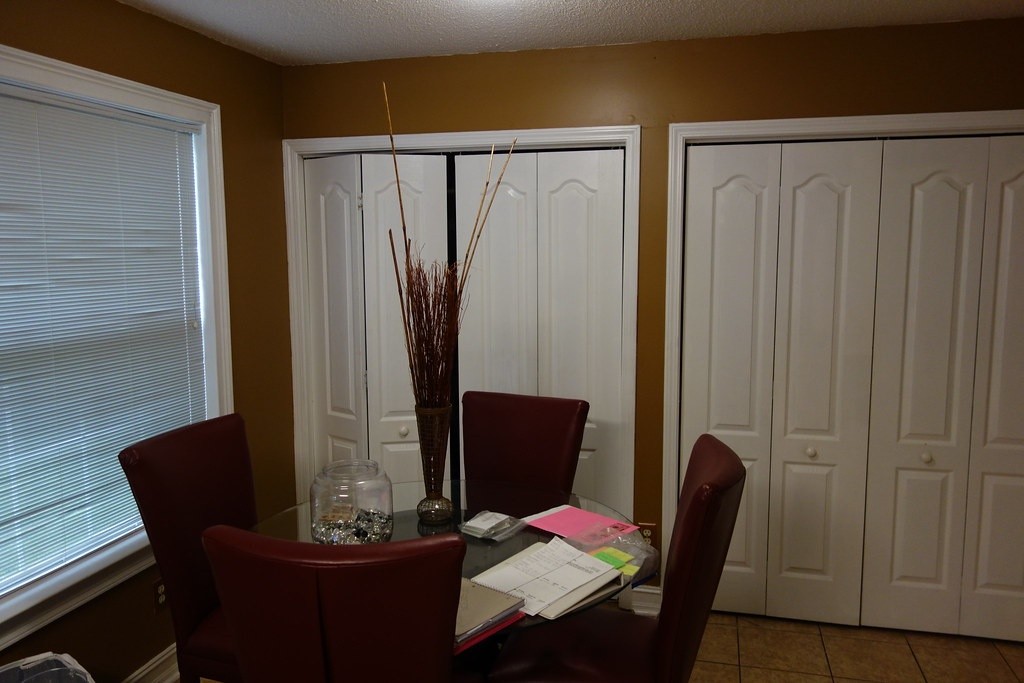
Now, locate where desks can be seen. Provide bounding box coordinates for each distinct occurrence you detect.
[256,481,659,683]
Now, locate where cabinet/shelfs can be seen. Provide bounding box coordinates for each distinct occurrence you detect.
[863,136,1024,642]
[679,140,884,626]
[454,149,624,525]
[296,151,447,505]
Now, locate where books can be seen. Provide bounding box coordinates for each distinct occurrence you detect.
[454,577,527,657]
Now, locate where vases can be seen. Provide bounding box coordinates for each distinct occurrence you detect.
[415,403,453,526]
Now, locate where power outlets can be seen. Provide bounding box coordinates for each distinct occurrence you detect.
[153,577,167,616]
[638,523,657,547]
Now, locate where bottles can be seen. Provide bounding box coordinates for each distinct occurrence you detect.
[309,458,393,545]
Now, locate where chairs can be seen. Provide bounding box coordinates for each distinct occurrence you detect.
[485,434,745,683]
[463,391,589,529]
[119,413,259,683]
[197,524,466,683]
